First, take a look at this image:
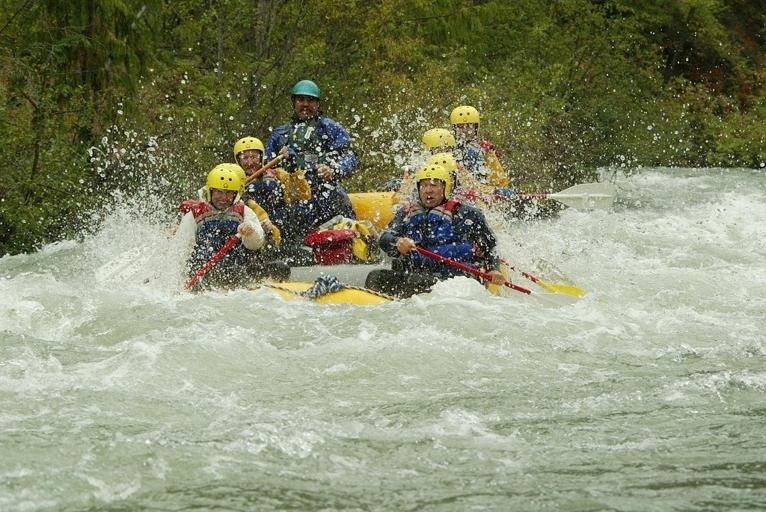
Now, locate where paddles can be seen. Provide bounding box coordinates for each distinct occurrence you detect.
[413,246,579,308]
[482,182,616,211]
[500,258,586,299]
[96,151,285,284]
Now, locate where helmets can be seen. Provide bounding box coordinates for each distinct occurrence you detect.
[416,105,480,202]
[206,80,321,204]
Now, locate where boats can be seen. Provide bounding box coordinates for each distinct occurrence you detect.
[246,259,597,331]
[274,181,591,247]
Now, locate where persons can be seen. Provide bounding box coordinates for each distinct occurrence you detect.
[400,129,503,199]
[365,165,506,298]
[177,168,290,288]
[266,79,360,219]
[449,106,529,201]
[391,151,492,214]
[217,163,313,266]
[234,136,324,236]
